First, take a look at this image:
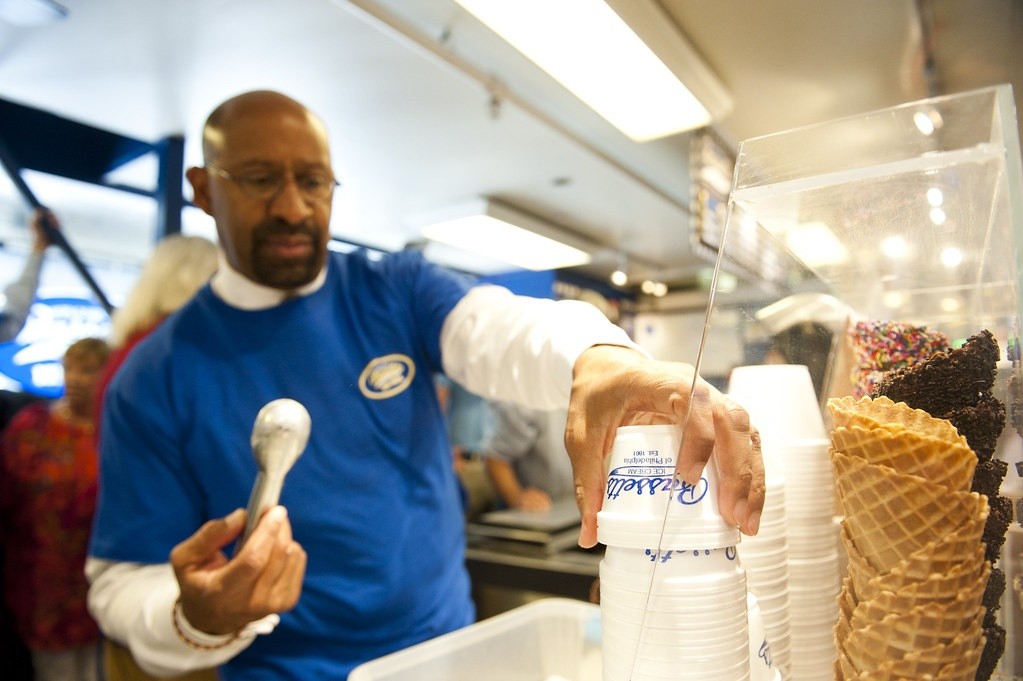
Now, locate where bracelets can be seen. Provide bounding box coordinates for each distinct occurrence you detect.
[172,597,238,651]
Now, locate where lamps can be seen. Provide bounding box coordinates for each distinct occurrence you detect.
[415,191,600,276]
[455,0,736,144]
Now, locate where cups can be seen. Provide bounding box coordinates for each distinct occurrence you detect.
[595,364,844,681]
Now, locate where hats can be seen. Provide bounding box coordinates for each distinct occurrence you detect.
[755,291,847,335]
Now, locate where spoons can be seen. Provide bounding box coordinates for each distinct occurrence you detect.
[232,398,312,559]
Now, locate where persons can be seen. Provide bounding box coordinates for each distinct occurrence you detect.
[434,372,499,524]
[755,291,849,400]
[487,267,642,516]
[82,90,766,679]
[0,208,222,681]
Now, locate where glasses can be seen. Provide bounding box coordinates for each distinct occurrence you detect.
[203,159,339,201]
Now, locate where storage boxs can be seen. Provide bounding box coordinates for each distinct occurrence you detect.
[620,82,1023,681]
[347,595,603,681]
